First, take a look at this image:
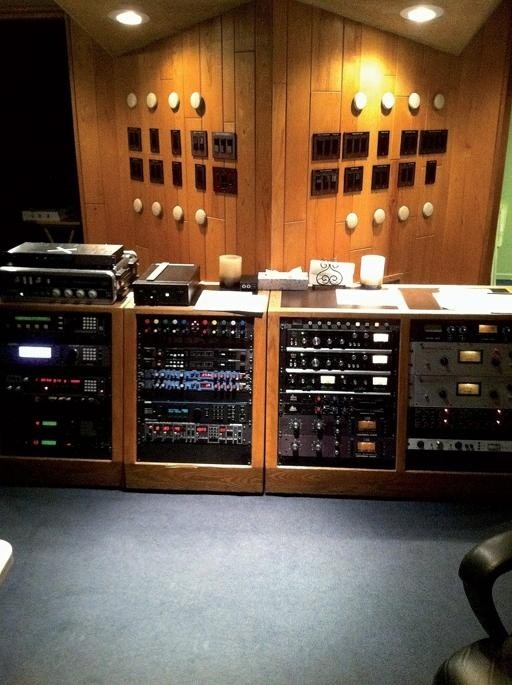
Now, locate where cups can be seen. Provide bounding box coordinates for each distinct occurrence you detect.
[358,254,386,289]
[219,254,242,286]
